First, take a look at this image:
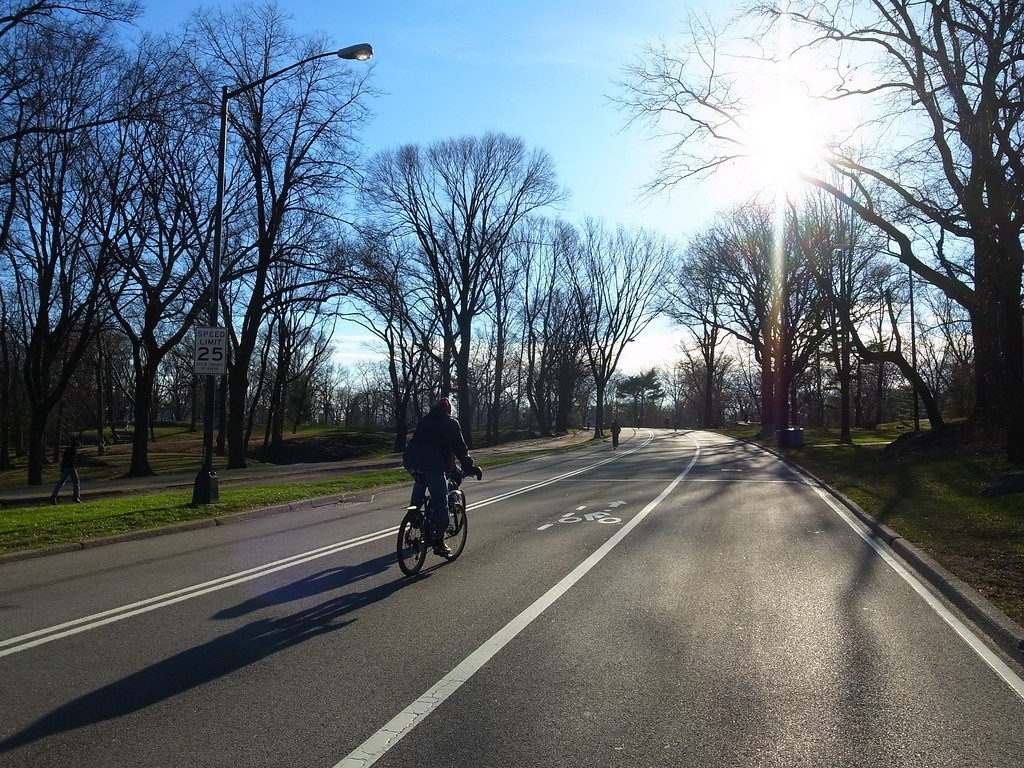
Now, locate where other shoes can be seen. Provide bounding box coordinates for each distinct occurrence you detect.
[409,521,419,529]
[433,541,451,553]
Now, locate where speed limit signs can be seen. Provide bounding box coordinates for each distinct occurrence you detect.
[193,325,229,375]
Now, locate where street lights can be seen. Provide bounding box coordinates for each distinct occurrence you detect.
[191,42,374,507]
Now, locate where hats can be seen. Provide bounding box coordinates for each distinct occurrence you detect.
[438,398,451,414]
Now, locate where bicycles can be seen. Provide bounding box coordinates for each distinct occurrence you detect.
[397,468,478,577]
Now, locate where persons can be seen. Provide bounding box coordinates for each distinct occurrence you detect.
[610,421,621,447]
[403,398,482,555]
[665,417,669,428]
[49,441,82,504]
